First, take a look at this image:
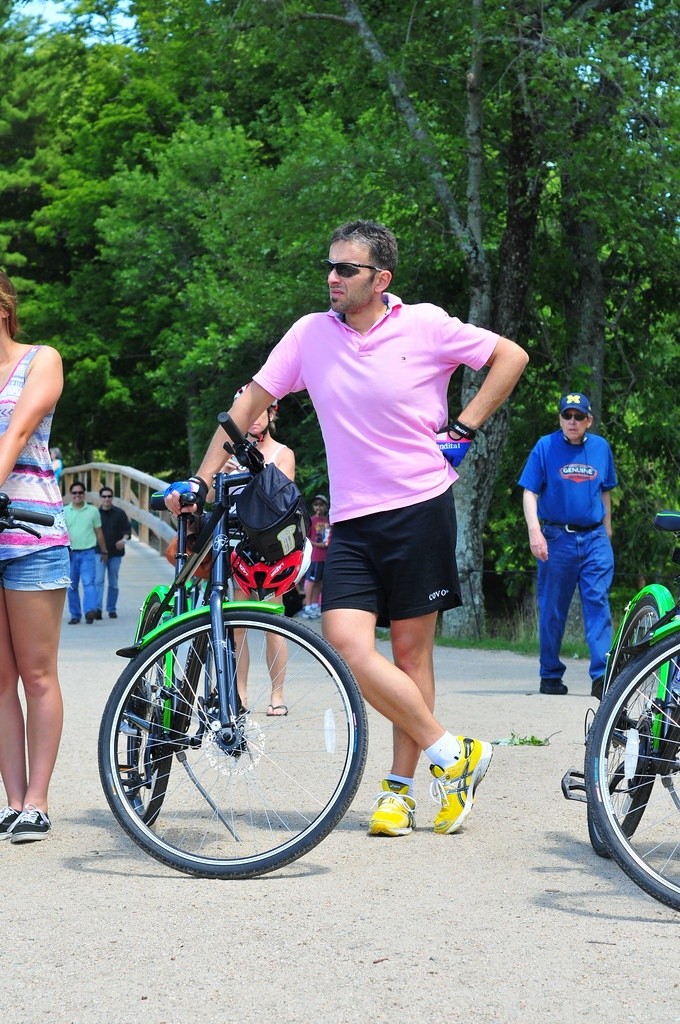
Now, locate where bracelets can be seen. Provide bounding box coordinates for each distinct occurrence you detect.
[122,538,128,543]
[101,551,109,555]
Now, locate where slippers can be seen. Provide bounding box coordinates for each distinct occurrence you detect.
[238,705,251,713]
[267,705,289,717]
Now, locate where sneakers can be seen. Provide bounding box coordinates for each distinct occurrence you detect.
[0,805,21,841]
[8,803,52,843]
[367,778,417,836]
[429,735,494,835]
[303,604,321,619]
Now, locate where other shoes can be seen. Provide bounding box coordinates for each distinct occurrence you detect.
[69,618,79,623]
[109,613,117,618]
[539,679,569,694]
[94,610,102,619]
[86,611,94,623]
[593,677,603,700]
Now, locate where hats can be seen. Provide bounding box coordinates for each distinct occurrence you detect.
[315,495,328,505]
[560,392,593,415]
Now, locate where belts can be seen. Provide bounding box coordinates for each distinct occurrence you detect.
[541,520,603,533]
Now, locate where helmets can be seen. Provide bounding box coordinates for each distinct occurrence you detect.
[232,535,312,604]
[233,382,279,412]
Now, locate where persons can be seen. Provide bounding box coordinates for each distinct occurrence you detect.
[49,447,64,487]
[204,381,295,716]
[165,220,530,836]
[0,271,70,846]
[64,482,132,626]
[518,393,618,701]
[301,495,331,619]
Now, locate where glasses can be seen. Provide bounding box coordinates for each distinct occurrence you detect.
[561,411,587,421]
[101,495,112,498]
[312,501,323,506]
[70,490,84,495]
[322,258,385,278]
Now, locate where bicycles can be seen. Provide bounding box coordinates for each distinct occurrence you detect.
[97,411,371,880]
[559,508,680,915]
